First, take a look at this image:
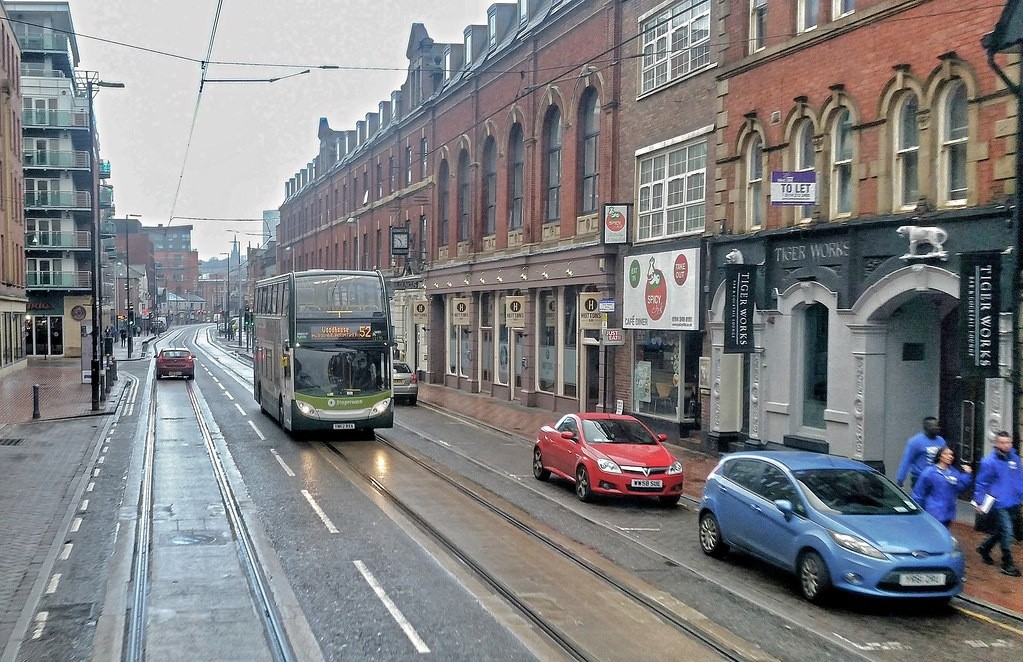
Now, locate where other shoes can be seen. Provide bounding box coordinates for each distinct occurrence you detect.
[1000,560,1022,576]
[976,544,994,565]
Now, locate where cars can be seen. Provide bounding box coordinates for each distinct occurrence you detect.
[697,451,967,612]
[532,412,685,509]
[154,348,196,380]
[393,358,418,405]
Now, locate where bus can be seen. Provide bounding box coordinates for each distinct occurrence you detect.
[249,266,395,438]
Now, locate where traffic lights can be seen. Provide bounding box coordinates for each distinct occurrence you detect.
[128,310,134,325]
[244,311,250,325]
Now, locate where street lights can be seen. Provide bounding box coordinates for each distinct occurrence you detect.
[124,214,143,357]
[220,250,231,341]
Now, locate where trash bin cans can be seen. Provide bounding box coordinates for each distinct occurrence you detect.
[104,337,114,357]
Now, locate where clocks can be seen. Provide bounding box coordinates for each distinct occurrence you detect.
[392,232,409,250]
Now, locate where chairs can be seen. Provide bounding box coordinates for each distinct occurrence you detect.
[647,382,674,415]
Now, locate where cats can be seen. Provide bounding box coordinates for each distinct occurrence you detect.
[895,225,948,254]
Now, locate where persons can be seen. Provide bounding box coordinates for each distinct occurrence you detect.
[973,431,1023,577]
[119,327,126,347]
[895,417,947,491]
[133,325,136,337]
[911,444,973,529]
[232,323,237,335]
[105,326,110,337]
[136,325,142,337]
[393,343,400,360]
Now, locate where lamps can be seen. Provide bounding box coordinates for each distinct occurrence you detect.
[421,278,428,290]
[479,271,486,286]
[433,277,439,289]
[422,327,430,332]
[463,328,474,334]
[517,331,530,339]
[463,273,470,286]
[564,262,575,279]
[542,264,549,281]
[496,270,504,285]
[446,275,452,288]
[520,266,529,281]
